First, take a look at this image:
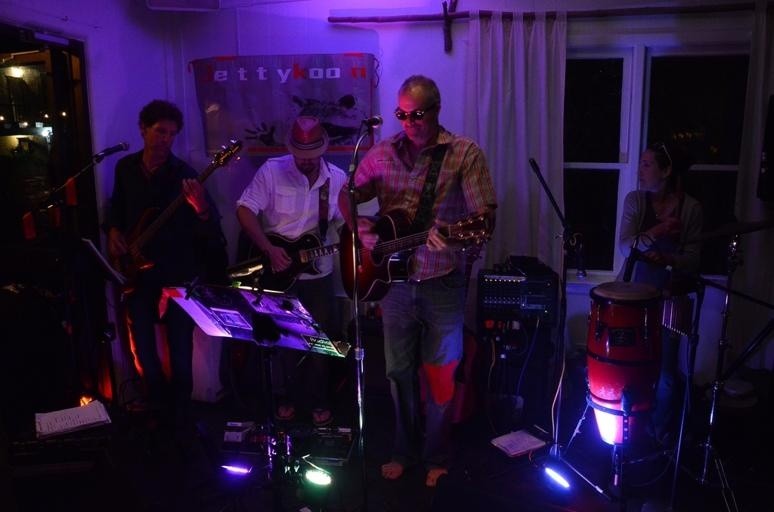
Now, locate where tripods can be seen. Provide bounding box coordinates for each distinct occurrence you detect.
[486,233,613,502]
[694,243,741,512]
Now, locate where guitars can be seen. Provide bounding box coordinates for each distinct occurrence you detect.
[249,231,341,292]
[338,210,490,302]
[113,138,244,287]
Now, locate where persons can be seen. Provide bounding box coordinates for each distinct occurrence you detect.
[338,76,498,485]
[618,133,711,286]
[95,97,226,400]
[234,116,348,428]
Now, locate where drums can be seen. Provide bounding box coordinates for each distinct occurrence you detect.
[587,284,659,444]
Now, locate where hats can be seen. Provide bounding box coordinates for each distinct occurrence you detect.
[285,115,330,160]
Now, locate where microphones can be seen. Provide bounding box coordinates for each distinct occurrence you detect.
[364,115,383,128]
[623,238,639,281]
[576,238,587,281]
[96,141,129,157]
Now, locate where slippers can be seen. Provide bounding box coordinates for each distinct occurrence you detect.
[312,407,333,428]
[274,404,296,421]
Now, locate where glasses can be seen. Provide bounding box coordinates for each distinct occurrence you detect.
[392,107,437,121]
[651,141,673,160]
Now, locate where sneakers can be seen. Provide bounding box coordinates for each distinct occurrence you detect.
[124,395,149,413]
[382,462,403,480]
[426,468,448,487]
